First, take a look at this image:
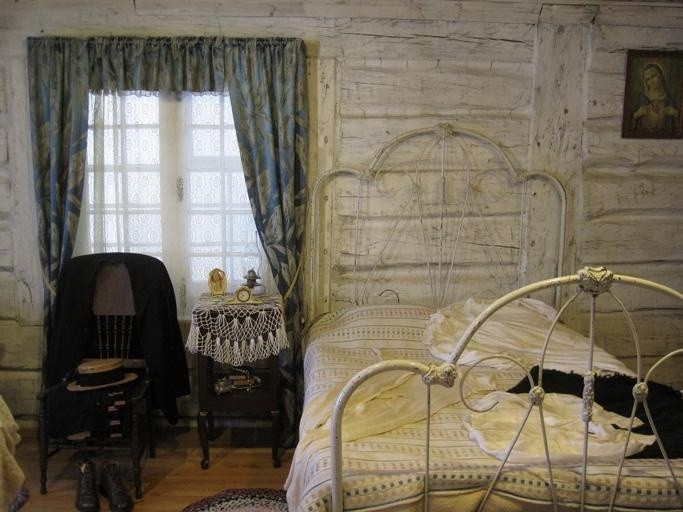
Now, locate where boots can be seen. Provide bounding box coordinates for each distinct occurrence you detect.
[75,459,132,512]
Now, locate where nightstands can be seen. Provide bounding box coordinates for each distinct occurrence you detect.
[199,294,284,468]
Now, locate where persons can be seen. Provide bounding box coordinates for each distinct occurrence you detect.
[628,63,681,134]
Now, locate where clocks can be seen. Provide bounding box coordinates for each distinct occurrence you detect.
[224,286,263,304]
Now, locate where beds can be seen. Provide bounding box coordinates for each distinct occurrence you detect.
[303,122,682,512]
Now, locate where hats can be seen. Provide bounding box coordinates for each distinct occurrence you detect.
[66,359,138,392]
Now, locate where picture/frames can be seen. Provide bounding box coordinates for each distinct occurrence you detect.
[621,48,683,140]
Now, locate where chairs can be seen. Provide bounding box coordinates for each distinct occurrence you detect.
[36,253,155,499]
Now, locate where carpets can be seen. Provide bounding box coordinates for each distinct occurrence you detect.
[183,489,291,512]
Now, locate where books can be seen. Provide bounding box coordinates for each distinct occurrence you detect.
[228,371,255,386]
